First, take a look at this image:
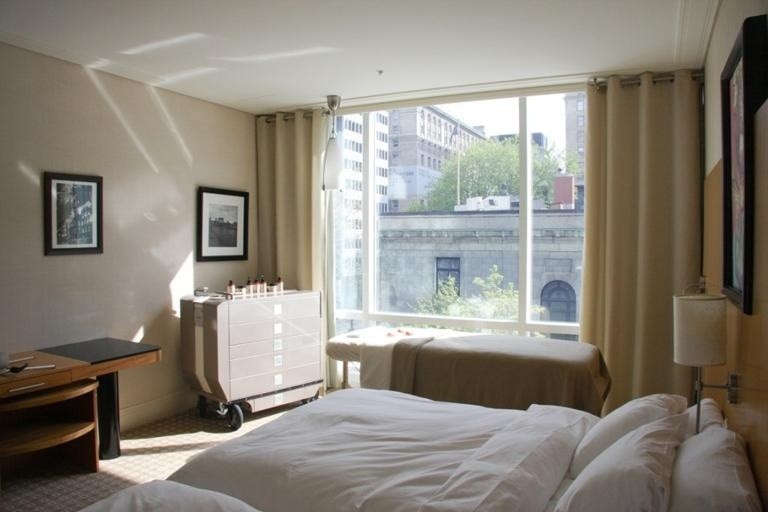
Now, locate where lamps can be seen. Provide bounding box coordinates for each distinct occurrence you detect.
[321,95,346,193]
[672,273,729,436]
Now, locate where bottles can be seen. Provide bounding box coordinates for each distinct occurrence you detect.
[227,276,283,294]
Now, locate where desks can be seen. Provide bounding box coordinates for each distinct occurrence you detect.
[0,338,162,476]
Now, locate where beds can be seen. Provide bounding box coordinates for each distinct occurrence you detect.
[327,324,610,415]
[77,479,257,511]
[167,386,718,511]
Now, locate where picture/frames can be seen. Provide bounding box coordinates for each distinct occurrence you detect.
[717,15,767,317]
[195,186,250,260]
[43,172,107,257]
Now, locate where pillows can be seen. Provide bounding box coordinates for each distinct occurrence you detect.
[570,392,689,479]
[554,412,688,512]
[669,425,762,512]
[683,398,725,435]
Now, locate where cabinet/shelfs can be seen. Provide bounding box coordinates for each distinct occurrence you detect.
[0,378,100,472]
